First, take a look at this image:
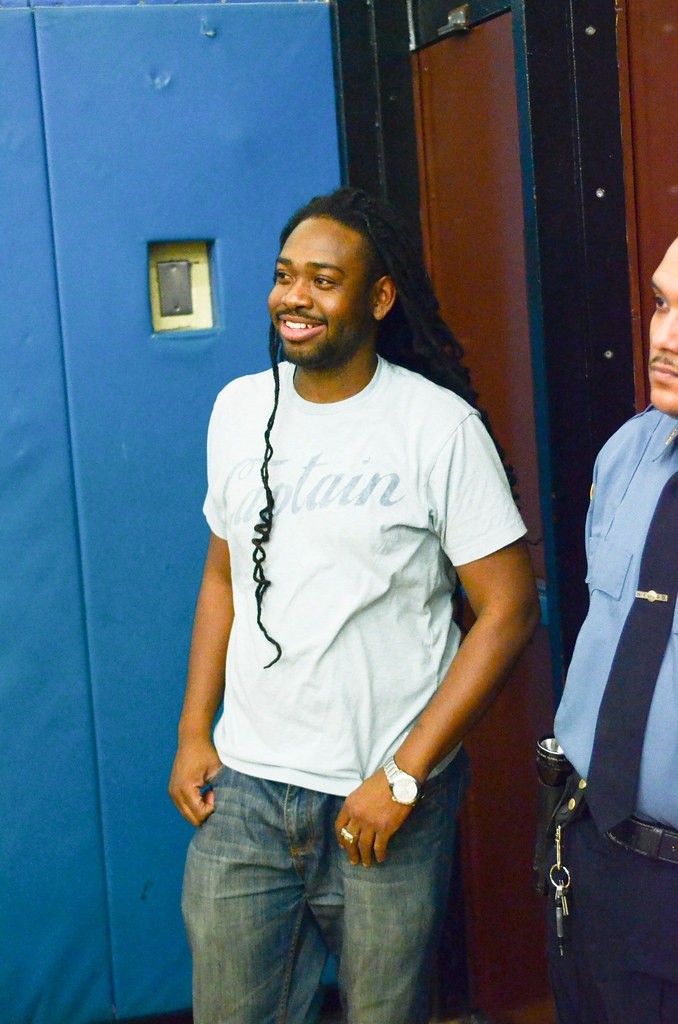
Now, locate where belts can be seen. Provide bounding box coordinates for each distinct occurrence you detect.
[607,817,678,864]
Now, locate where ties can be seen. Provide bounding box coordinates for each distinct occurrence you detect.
[582,471,678,836]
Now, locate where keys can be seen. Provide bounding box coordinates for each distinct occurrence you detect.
[549,863,571,960]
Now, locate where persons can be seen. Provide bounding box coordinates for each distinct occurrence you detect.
[170,185,542,1024]
[544,243,678,1024]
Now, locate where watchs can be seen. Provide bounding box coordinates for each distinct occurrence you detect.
[383,757,424,808]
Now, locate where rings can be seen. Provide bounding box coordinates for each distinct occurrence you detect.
[339,829,359,843]
